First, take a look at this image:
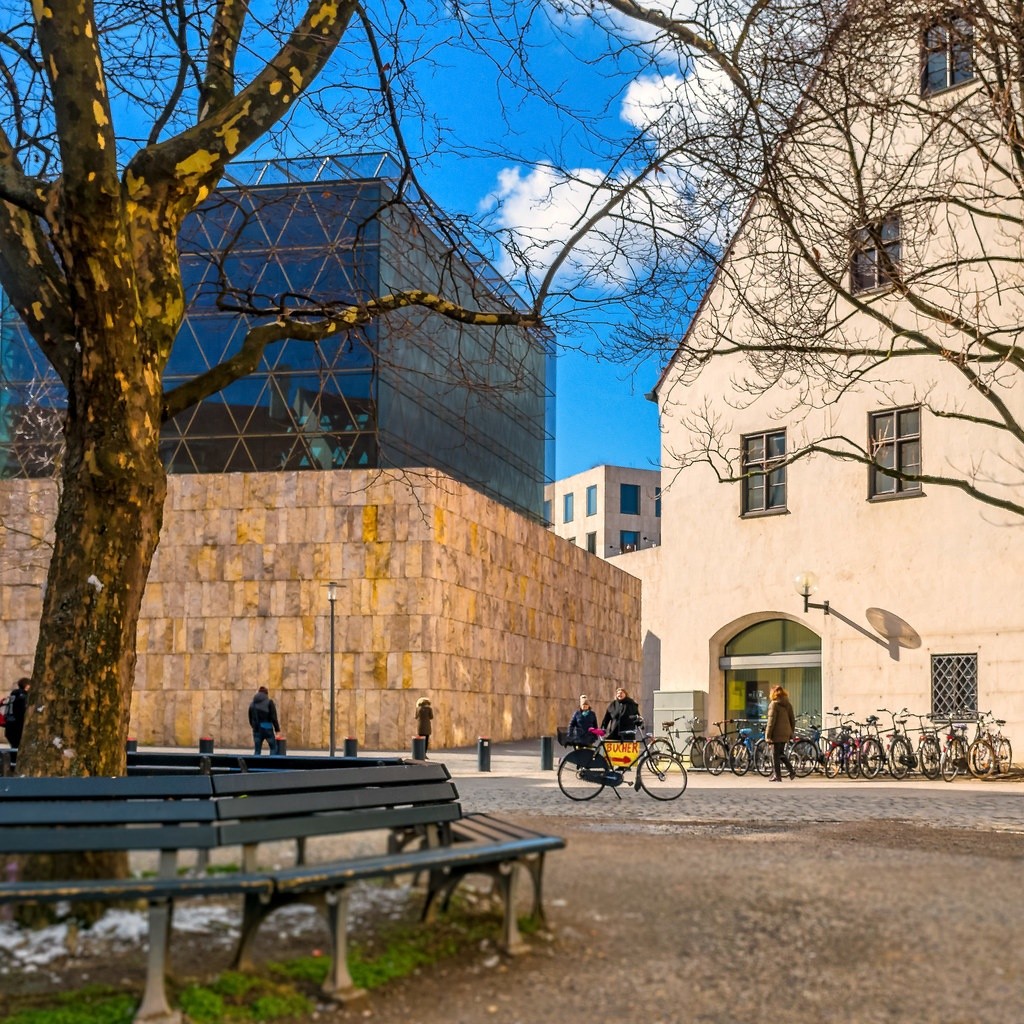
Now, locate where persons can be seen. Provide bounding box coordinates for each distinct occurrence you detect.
[248,686,281,755]
[4,677,31,748]
[746,696,763,719]
[415,697,433,759]
[765,686,796,782]
[601,688,640,771]
[567,694,598,779]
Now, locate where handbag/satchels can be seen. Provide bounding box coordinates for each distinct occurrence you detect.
[258,727,274,738]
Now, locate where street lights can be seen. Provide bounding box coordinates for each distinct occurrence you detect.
[319,581,347,755]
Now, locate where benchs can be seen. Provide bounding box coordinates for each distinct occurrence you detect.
[0,735,566,1023]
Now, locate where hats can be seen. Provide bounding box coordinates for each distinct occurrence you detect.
[579,694,591,708]
[258,686,268,695]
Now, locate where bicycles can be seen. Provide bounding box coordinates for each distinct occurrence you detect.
[729,707,996,773]
[645,716,712,775]
[964,706,1012,777]
[877,707,914,779]
[557,715,687,801]
[702,721,738,776]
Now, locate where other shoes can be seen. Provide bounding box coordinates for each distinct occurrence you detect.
[425,756,428,759]
[627,768,631,771]
[615,766,624,771]
[576,766,580,770]
[790,769,796,780]
[768,774,782,782]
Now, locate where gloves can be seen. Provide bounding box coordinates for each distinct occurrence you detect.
[563,736,572,749]
[591,736,598,742]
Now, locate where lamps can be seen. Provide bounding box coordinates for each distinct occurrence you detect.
[793,570,829,615]
[642,536,656,547]
[609,545,622,554]
